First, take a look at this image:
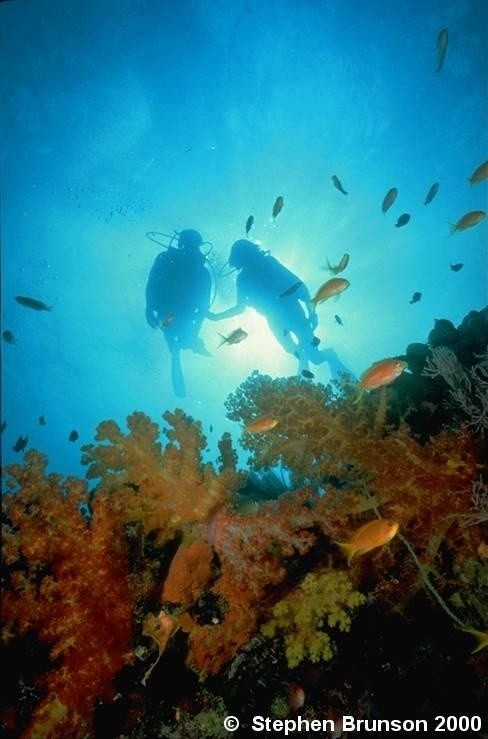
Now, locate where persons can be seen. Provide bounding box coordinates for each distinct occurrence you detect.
[206,239,357,385]
[145,230,213,396]
